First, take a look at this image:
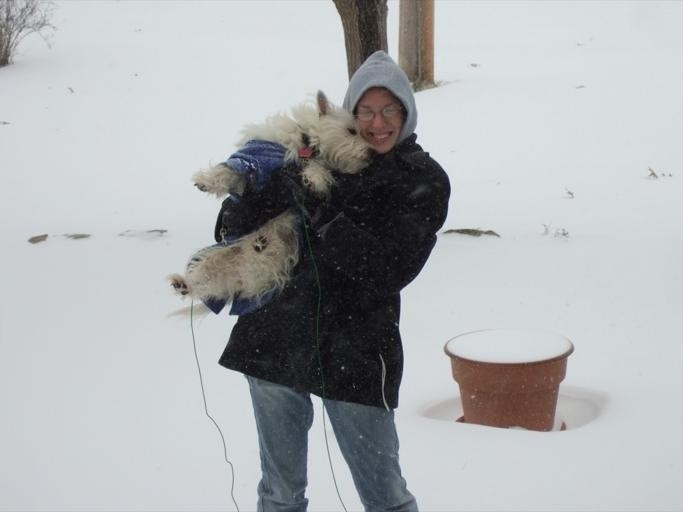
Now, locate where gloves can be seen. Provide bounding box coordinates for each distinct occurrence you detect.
[220,199,251,236]
[292,183,338,224]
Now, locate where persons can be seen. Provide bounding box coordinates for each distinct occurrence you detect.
[216,44,452,512]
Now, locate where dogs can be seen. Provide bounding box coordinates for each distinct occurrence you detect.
[166,89,375,313]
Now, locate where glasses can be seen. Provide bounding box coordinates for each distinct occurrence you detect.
[354,103,404,125]
